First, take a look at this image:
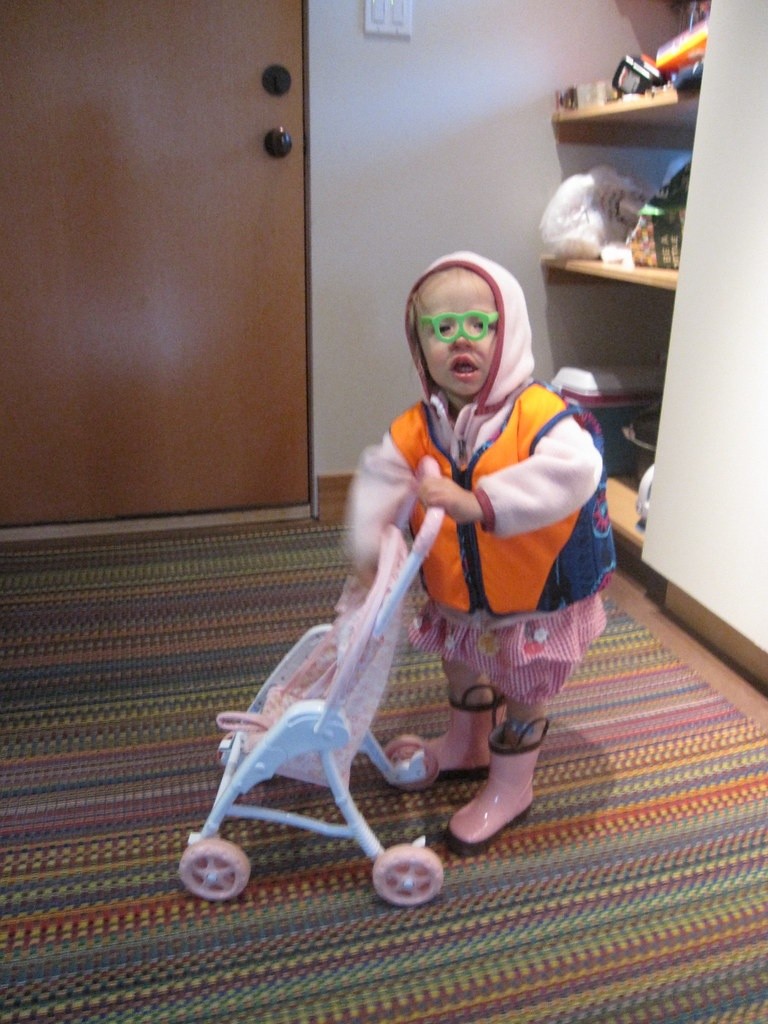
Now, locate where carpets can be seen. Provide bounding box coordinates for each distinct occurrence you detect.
[0,515,768,1024]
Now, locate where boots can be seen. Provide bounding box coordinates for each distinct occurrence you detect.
[445,718,550,855]
[426,684,506,783]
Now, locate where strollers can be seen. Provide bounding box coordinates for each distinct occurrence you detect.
[176,454,446,907]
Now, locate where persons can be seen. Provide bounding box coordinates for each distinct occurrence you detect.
[340,254,620,858]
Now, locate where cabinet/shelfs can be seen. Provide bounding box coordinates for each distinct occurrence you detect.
[539,84,702,608]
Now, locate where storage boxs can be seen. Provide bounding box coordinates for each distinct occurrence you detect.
[550,363,665,478]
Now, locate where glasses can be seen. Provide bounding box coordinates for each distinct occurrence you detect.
[419,311,499,343]
